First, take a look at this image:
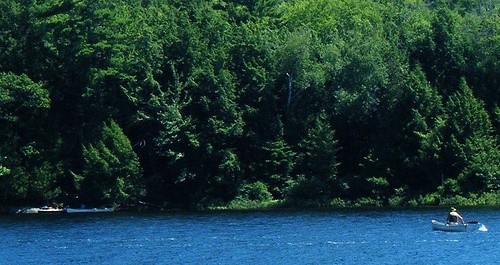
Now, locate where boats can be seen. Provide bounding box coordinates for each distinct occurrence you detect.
[431,219,482,232]
[38,207,114,213]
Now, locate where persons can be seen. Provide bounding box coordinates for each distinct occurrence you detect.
[446,207,464,225]
